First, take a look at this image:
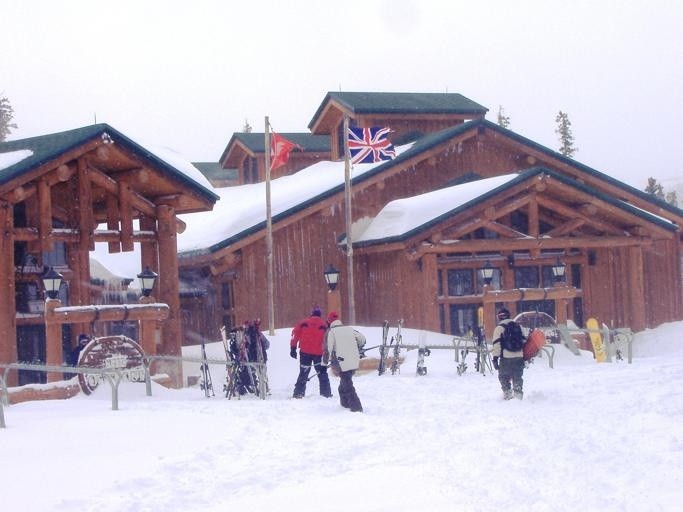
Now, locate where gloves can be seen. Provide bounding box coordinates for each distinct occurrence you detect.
[290,347,297,358]
[492,359,498,370]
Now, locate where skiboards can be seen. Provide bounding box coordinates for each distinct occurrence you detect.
[201,332,208,396]
[379,319,390,376]
[416,330,426,375]
[392,319,404,374]
[457,323,487,375]
[219,319,271,400]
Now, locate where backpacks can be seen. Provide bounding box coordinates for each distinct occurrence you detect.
[496,321,524,352]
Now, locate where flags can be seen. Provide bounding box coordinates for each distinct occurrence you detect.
[347,126,396,164]
[269,133,305,169]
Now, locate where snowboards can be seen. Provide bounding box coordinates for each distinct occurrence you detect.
[602,322,612,362]
[612,330,624,363]
[557,323,579,355]
[586,318,605,363]
[523,328,546,361]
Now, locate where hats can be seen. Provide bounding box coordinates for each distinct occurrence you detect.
[327,310,339,325]
[498,310,510,320]
[310,307,321,316]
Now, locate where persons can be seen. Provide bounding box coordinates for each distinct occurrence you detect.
[461,326,473,339]
[289,304,333,399]
[69,334,90,368]
[491,308,528,401]
[319,311,366,413]
